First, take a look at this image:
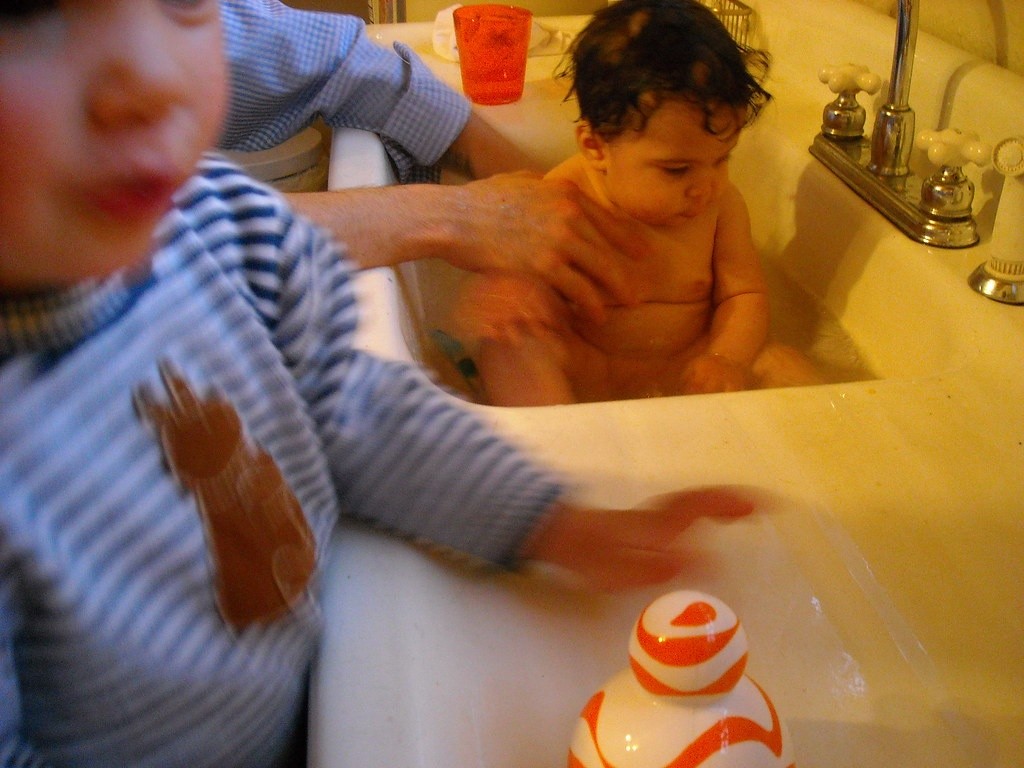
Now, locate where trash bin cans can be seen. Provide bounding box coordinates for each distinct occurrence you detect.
[226,125,329,196]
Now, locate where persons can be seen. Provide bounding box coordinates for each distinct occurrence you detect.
[0,0,825,768]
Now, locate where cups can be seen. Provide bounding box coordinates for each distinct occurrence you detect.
[454,6,531,105]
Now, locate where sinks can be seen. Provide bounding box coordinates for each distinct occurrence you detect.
[328,88,889,440]
[312,440,1024,767]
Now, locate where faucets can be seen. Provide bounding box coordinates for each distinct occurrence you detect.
[865,0,919,178]
